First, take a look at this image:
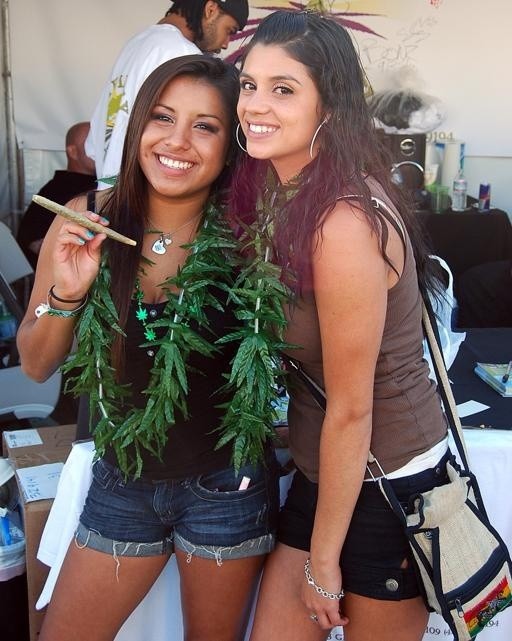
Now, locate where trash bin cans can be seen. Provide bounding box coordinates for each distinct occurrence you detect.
[0,458,30,641]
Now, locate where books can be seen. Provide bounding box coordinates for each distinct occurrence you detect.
[474,363,511,397]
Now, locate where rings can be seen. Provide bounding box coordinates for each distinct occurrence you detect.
[311,615,316,620]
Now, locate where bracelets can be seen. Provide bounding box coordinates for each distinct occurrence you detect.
[305,557,345,600]
[49,285,86,302]
[34,293,82,319]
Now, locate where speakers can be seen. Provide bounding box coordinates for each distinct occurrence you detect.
[378,132,427,211]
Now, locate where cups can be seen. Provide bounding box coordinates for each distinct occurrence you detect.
[426,184,449,213]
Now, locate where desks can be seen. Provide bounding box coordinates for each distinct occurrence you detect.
[3,425,78,640]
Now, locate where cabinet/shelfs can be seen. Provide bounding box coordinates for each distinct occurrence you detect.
[409,188,511,326]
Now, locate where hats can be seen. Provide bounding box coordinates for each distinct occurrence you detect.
[213,0,249,30]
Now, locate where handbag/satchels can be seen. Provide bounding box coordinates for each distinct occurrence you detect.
[405,454,512,641]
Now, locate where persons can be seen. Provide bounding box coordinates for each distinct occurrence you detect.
[15,1,250,261]
[15,54,279,640]
[236,1,465,640]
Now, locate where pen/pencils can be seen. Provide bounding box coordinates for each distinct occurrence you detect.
[502,360,512,383]
